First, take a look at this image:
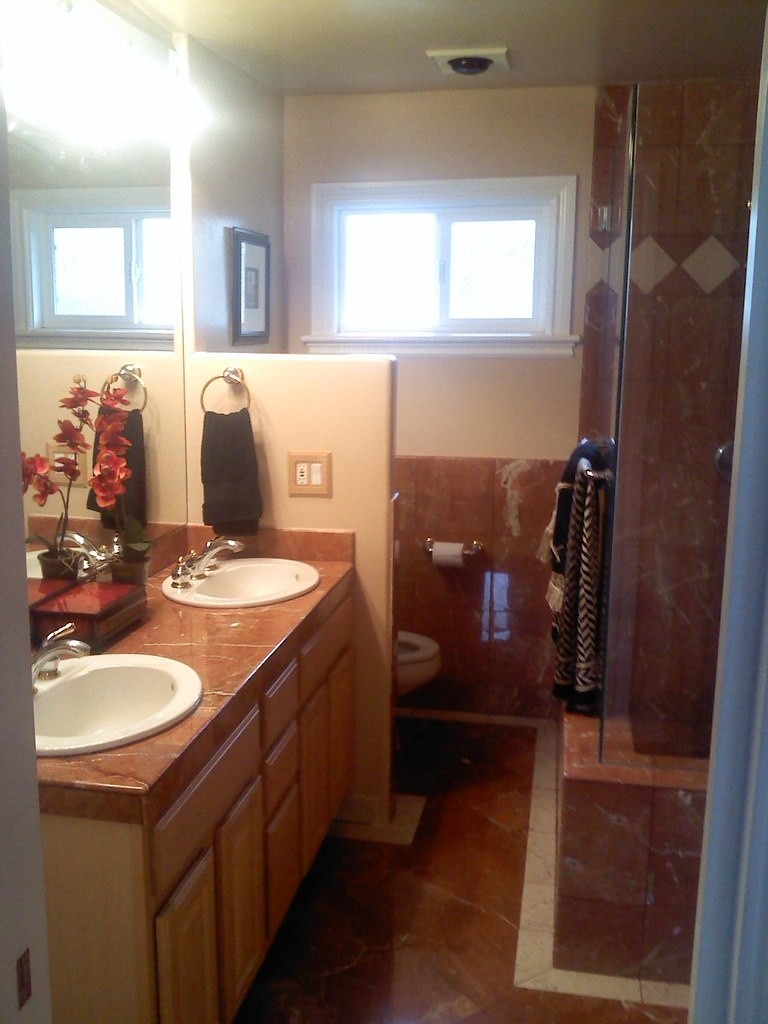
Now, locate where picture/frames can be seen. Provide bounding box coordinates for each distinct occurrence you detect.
[231,223,274,343]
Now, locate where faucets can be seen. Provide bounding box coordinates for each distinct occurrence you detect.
[57,530,107,568]
[190,539,244,580]
[30,639,92,695]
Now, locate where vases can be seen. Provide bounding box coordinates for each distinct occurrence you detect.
[39,545,81,578]
[106,556,153,594]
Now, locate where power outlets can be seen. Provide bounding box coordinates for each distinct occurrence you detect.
[287,450,333,501]
[16,948,32,1011]
[46,440,88,489]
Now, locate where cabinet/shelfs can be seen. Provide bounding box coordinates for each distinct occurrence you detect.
[41,622,374,1024]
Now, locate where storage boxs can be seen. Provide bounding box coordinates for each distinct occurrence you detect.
[36,580,148,653]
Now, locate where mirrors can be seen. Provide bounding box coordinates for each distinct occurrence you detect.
[11,5,194,610]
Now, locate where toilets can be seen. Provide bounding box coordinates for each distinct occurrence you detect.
[396,630,444,692]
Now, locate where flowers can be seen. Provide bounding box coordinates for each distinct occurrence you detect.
[93,370,144,548]
[21,375,104,555]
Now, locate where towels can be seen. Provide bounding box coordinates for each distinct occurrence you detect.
[200,408,263,537]
[550,440,607,706]
[85,406,147,529]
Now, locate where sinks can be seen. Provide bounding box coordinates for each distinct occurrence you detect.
[162,557,321,608]
[30,652,204,757]
[23,547,110,579]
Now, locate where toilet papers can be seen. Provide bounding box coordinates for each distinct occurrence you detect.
[432,541,467,568]
